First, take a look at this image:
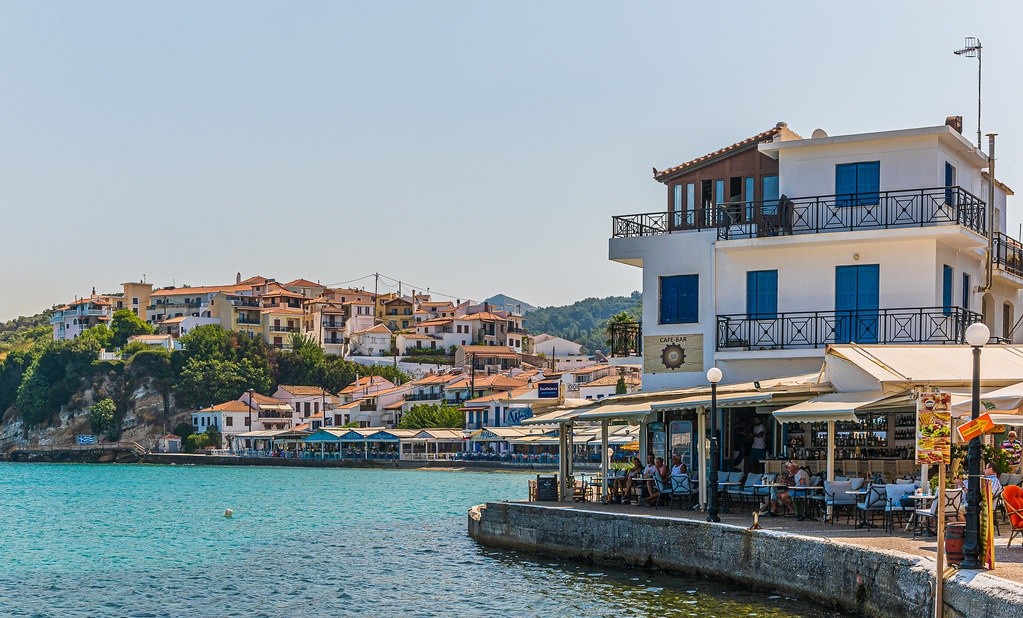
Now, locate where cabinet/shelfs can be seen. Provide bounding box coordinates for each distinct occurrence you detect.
[783,412,915,460]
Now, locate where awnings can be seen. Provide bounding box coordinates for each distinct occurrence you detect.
[577,402,654,424]
[522,410,589,426]
[651,392,773,412]
[772,392,899,425]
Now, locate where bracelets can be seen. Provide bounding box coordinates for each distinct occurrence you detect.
[962,485,965,490]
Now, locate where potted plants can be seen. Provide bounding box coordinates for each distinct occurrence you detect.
[605,310,639,358]
[565,473,576,501]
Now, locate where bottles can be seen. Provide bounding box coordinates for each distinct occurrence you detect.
[788,413,916,461]
[762,478,765,485]
[926,481,932,495]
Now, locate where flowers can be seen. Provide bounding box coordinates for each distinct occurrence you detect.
[984,443,1020,474]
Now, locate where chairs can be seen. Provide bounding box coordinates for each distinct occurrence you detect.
[566,468,1023,549]
[754,194,795,235]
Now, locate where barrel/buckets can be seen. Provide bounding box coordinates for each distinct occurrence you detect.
[945,522,966,565]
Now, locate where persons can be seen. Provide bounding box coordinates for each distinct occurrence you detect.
[948,463,1001,522]
[1003,431,1023,474]
[482,443,487,453]
[272,446,284,457]
[608,453,687,504]
[752,416,766,474]
[766,460,811,517]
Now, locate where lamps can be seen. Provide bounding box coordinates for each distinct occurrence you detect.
[907,495,937,537]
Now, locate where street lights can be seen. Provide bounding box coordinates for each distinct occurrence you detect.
[957,321,992,570]
[705,367,723,522]
[608,447,614,469]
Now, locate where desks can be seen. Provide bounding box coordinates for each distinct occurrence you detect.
[631,477,654,506]
[718,482,741,514]
[844,492,869,528]
[788,486,823,521]
[752,484,786,518]
[591,477,615,480]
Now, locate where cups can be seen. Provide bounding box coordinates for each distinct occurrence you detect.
[766,479,769,485]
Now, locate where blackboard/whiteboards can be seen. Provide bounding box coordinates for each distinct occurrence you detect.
[537,473,558,501]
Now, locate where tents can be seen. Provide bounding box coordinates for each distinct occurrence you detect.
[511,436,634,461]
[950,383,1023,466]
